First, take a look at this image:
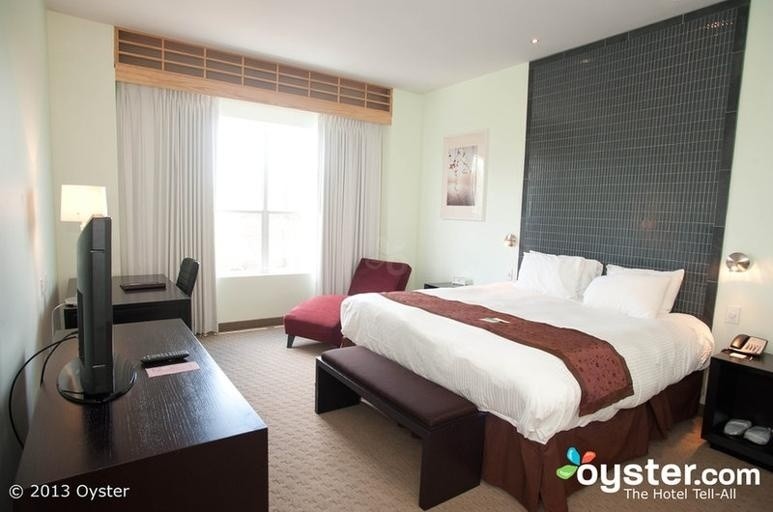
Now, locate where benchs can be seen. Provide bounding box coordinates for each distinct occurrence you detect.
[315,346,487,511]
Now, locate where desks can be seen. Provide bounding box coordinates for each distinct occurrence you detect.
[63,275,191,330]
[11,319,268,512]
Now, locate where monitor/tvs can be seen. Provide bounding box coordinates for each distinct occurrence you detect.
[58,214,137,407]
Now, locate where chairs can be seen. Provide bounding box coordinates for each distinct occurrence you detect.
[284,258,412,349]
[176,258,199,331]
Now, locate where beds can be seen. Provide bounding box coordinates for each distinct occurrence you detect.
[340,279,714,512]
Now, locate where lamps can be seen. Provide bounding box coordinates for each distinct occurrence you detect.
[61,185,107,230]
[727,253,751,273]
[505,234,516,247]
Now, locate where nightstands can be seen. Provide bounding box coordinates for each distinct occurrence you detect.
[700,349,773,473]
[423,282,463,289]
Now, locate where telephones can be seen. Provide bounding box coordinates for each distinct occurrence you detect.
[720,334,768,358]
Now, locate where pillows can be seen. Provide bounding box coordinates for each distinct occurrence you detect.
[517,252,584,300]
[530,249,604,289]
[584,276,669,319]
[607,264,685,313]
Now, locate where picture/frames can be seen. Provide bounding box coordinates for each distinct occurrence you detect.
[440,132,488,222]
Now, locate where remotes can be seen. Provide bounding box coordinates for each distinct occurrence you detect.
[141,347,192,371]
